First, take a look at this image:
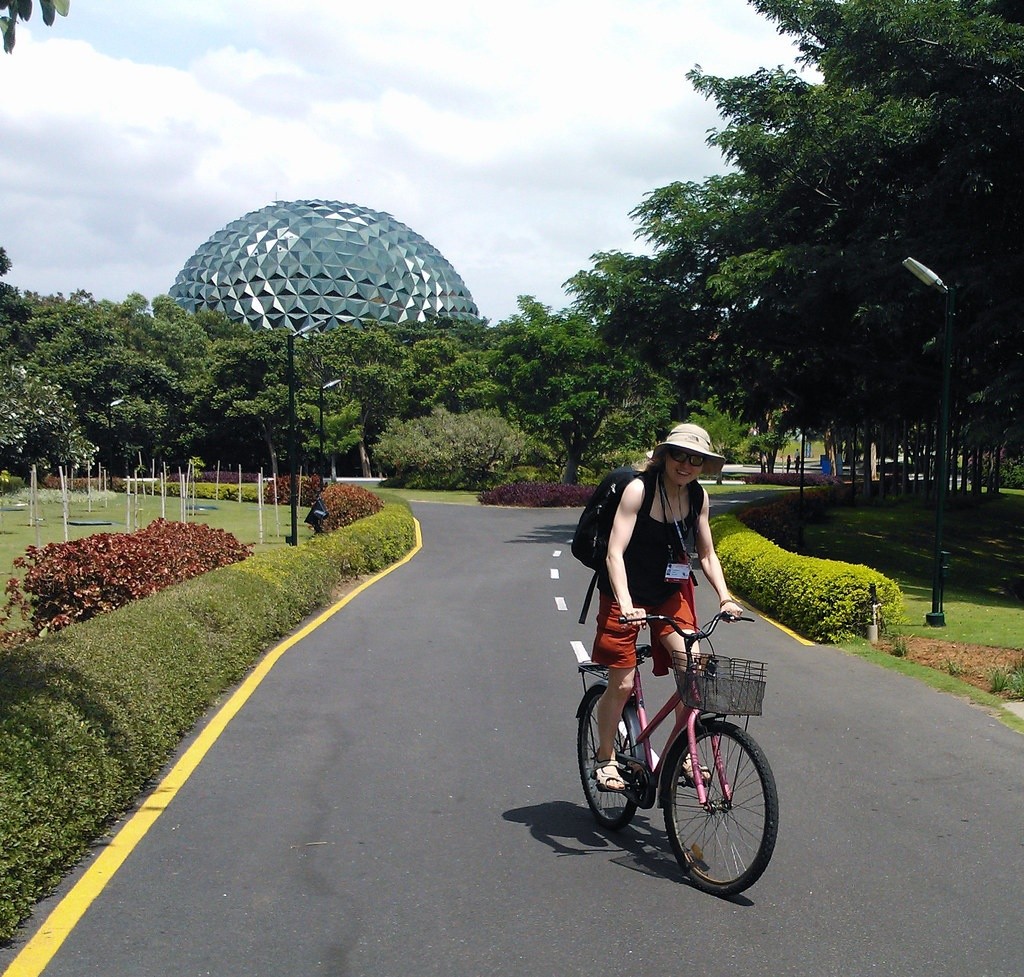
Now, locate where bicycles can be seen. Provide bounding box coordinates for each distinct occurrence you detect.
[576,610,779,898]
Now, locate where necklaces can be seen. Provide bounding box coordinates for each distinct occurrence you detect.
[591,422,745,791]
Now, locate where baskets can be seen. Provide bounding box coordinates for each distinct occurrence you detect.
[672,650,768,716]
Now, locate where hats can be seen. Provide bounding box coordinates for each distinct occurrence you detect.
[651,423,725,475]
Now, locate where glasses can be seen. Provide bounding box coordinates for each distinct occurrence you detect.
[666,446,707,467]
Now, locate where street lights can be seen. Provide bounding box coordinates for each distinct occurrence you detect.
[107,399,124,491]
[902,256,950,629]
[286,318,328,545]
[318,380,340,496]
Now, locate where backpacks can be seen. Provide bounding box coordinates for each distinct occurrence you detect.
[571,468,654,624]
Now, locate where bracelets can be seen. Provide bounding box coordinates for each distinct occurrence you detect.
[720,599,735,608]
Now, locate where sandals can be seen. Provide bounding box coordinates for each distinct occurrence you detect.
[590,757,625,793]
[681,753,712,782]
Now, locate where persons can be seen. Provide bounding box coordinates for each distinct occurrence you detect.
[795,449,799,459]
[793,455,800,474]
[786,455,791,473]
